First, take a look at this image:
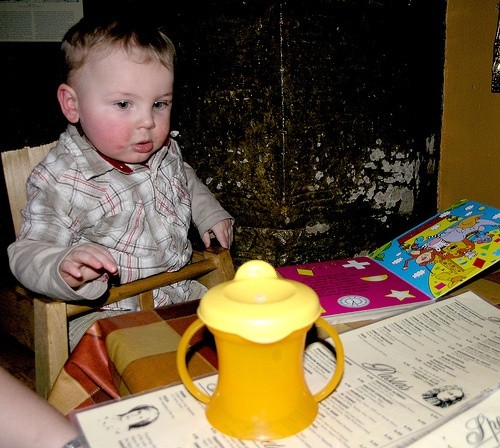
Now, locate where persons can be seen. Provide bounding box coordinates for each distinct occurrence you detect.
[6,9,235,355]
[0,367,91,448]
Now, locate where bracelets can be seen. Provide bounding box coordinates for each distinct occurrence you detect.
[60,435,87,448]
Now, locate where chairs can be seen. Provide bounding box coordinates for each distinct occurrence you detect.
[1,139,237,403]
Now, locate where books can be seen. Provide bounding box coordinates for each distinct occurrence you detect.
[275,198,500,325]
[70,289,500,447]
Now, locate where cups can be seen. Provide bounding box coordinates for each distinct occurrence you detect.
[177,260,344,441]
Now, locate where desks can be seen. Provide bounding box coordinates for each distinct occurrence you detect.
[99,264,500,396]
